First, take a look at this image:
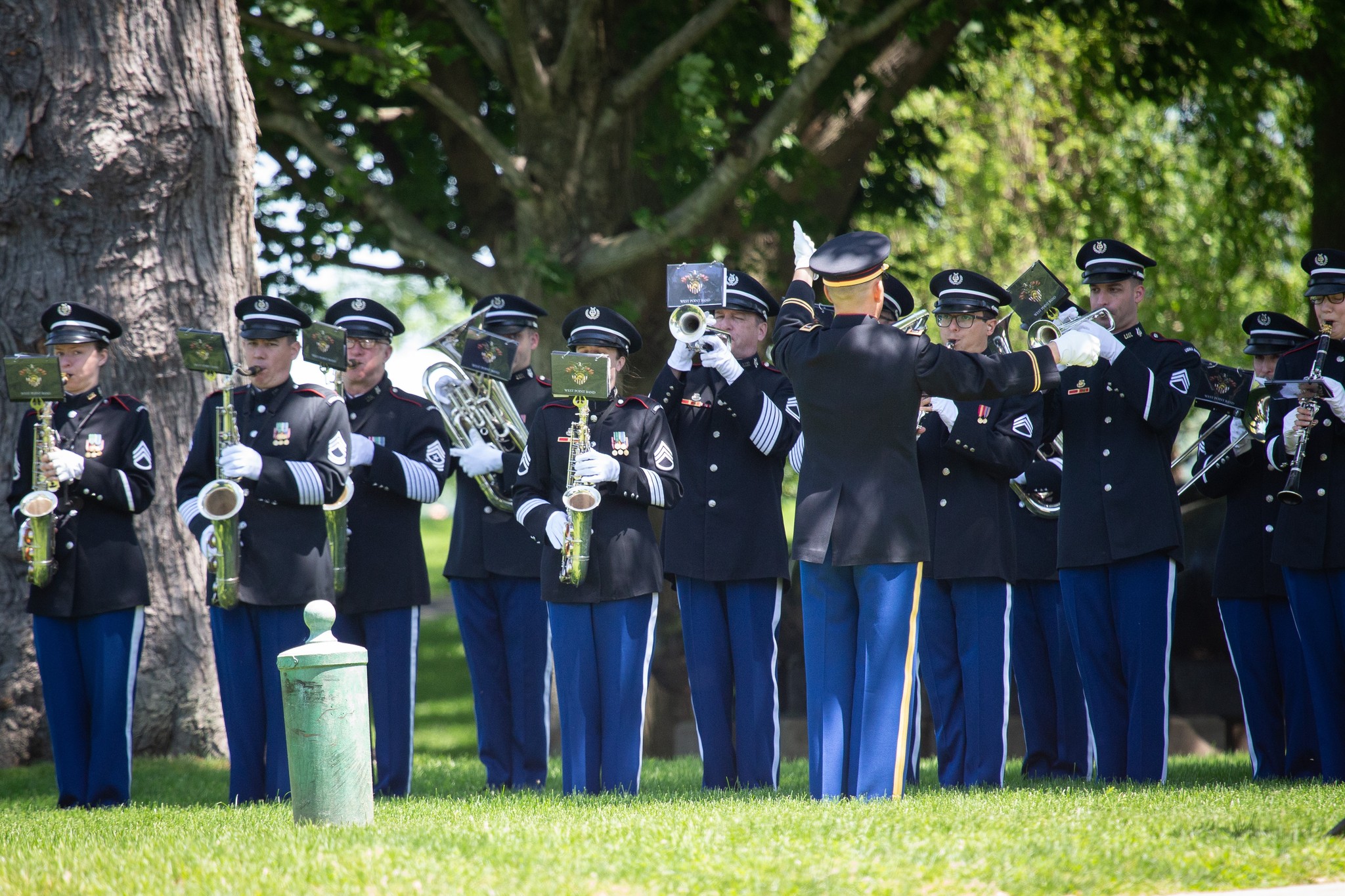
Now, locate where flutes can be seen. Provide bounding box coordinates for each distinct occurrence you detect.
[916,338,956,435]
[1276,319,1334,507]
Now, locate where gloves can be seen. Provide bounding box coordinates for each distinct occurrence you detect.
[435,374,473,417]
[792,220,820,280]
[1070,319,1125,366]
[545,511,594,551]
[1013,472,1027,486]
[199,521,247,567]
[1230,416,1252,456]
[574,446,621,484]
[699,333,745,386]
[667,311,717,372]
[218,442,263,481]
[349,432,374,467]
[1050,329,1101,367]
[449,427,504,478]
[1052,307,1079,327]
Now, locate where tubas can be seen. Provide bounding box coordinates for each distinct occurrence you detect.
[416,303,530,514]
[986,309,1065,521]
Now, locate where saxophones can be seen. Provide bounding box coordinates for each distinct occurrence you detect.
[18,372,75,590]
[322,357,360,597]
[559,399,602,588]
[197,363,263,612]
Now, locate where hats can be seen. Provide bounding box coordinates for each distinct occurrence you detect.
[1242,311,1317,356]
[929,268,1012,316]
[40,301,123,346]
[325,297,405,343]
[234,295,312,339]
[1076,239,1157,285]
[722,269,781,322]
[561,306,643,356]
[1301,248,1345,297]
[471,293,548,335]
[881,272,915,321]
[809,231,892,287]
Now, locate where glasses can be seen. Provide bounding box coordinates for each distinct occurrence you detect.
[935,313,989,329]
[1308,292,1345,304]
[345,337,389,350]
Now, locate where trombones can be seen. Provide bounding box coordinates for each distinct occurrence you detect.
[1170,375,1273,497]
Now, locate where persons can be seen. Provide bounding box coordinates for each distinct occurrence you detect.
[647,269,803,791]
[512,305,685,796]
[768,221,1345,804]
[324,298,451,799]
[175,295,351,804]
[435,294,569,791]
[9,301,156,809]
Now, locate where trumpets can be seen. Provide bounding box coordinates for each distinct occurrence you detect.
[1027,307,1116,349]
[669,303,732,354]
